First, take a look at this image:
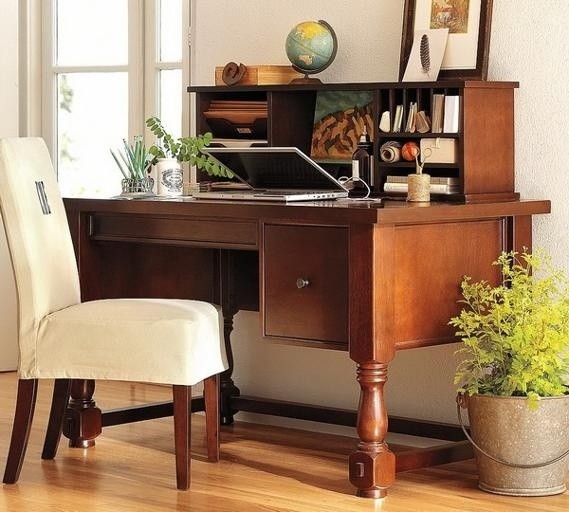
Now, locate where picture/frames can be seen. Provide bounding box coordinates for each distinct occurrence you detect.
[397,0,496,84]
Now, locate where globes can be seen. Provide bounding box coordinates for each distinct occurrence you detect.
[284,19,338,85]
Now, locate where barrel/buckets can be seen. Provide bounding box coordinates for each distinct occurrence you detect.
[452,387,569,495]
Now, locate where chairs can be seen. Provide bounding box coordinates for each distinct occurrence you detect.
[1,136,229,491]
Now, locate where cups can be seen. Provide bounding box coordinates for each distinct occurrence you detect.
[407,174,430,203]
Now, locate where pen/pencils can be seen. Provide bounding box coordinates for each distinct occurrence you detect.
[109,135,159,191]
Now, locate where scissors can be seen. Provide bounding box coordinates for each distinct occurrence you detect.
[410,146,432,174]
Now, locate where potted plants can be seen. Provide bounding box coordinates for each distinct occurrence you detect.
[109,110,233,198]
[447,242,569,500]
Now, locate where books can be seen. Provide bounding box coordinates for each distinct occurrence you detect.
[384,182,461,194]
[432,94,445,134]
[406,101,417,133]
[392,103,403,132]
[387,174,460,184]
[443,95,458,133]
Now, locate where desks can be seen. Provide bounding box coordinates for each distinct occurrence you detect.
[55,190,553,500]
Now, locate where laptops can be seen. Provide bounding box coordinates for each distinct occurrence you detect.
[191,145,350,201]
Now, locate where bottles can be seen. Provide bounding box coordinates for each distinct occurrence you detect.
[351,124,375,192]
